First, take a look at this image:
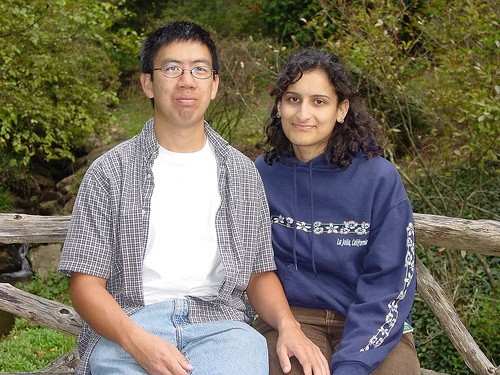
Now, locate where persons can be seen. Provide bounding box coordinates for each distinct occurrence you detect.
[57,14,332,375]
[254,44,422,375]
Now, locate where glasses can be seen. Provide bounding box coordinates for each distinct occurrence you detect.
[150,65,217,79]
